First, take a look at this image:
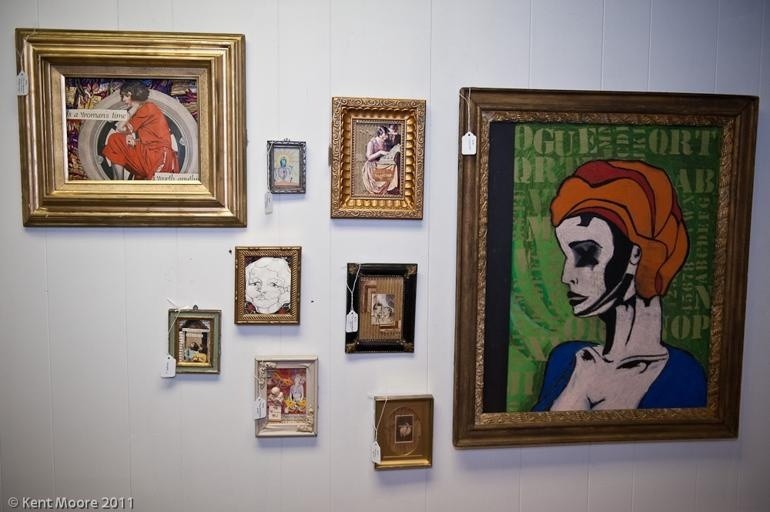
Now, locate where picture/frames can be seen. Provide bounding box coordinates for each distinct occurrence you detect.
[344,262,417,354]
[331,96,426,220]
[266,138,307,194]
[370,396,434,470]
[167,307,222,376]
[452,86,761,449]
[234,246,303,325]
[15,27,248,230]
[255,358,320,437]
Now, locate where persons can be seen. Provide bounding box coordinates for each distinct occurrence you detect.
[274,156,292,182]
[290,374,304,401]
[268,386,284,407]
[373,295,393,323]
[104,79,180,181]
[184,342,199,361]
[362,124,401,195]
